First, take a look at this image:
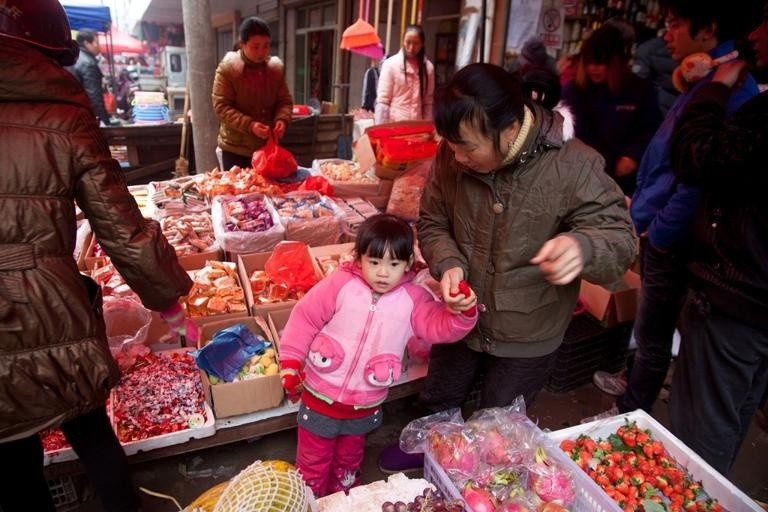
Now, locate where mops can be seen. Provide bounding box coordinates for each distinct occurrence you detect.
[362,0,381,110]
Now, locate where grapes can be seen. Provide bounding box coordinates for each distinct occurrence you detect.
[383,486,466,512]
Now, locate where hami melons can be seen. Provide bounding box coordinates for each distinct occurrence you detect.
[181,459,313,512]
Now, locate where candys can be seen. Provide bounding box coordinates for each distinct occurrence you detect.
[106,351,206,443]
[40,428,70,450]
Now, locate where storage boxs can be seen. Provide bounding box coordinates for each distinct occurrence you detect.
[578,269,640,326]
[544,408,767,512]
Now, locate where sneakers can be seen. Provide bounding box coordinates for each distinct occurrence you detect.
[591,368,630,395]
[378,442,425,473]
[580,407,620,424]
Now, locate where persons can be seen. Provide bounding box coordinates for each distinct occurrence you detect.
[673,3,768,474]
[376,62,637,473]
[211,16,293,170]
[516,20,682,196]
[372,24,436,123]
[67,27,112,129]
[0,0,194,512]
[279,214,480,500]
[578,0,761,425]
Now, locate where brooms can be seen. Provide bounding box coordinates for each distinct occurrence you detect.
[173,84,190,177]
[339,0,384,64]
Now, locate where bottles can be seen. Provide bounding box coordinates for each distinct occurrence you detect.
[560,0,664,58]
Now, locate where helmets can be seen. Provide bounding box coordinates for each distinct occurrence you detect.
[0,0,80,66]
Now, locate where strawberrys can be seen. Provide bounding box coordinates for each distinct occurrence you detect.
[560,417,725,512]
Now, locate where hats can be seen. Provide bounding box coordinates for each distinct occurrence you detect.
[521,36,544,65]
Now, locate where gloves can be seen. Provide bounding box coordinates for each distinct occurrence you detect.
[449,281,476,317]
[277,360,305,394]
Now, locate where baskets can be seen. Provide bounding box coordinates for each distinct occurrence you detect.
[544,322,634,395]
[43,475,95,510]
